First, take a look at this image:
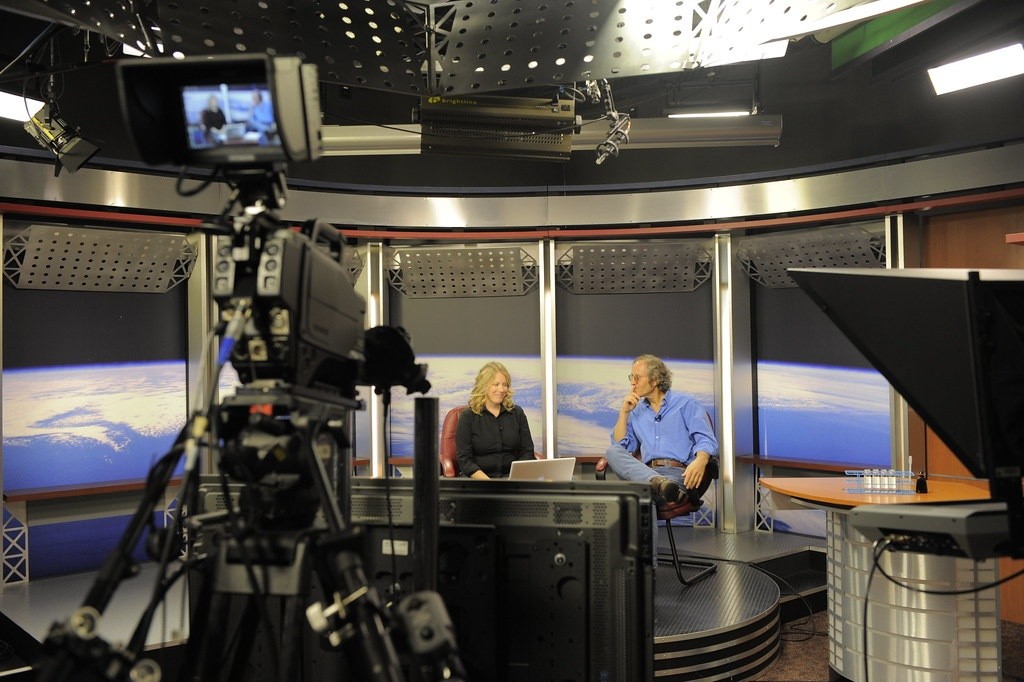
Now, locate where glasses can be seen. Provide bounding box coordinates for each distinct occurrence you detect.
[629,374,649,382]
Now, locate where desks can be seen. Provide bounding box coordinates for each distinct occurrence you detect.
[760,477,1002,682]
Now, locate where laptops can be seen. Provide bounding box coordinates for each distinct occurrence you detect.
[509,457,576,481]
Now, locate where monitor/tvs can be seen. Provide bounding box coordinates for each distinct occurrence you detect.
[190,475,653,682]
[421,83,576,160]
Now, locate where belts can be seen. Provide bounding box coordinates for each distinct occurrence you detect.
[648,460,687,468]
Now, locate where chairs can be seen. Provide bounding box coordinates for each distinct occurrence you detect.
[441,407,545,478]
[595,412,718,585]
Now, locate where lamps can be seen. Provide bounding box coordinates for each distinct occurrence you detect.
[663,106,752,118]
[808,19,865,47]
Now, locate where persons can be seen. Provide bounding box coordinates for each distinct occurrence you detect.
[605,355,720,571]
[454,362,538,479]
[200,88,278,146]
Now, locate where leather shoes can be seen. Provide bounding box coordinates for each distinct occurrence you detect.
[649,476,680,503]
